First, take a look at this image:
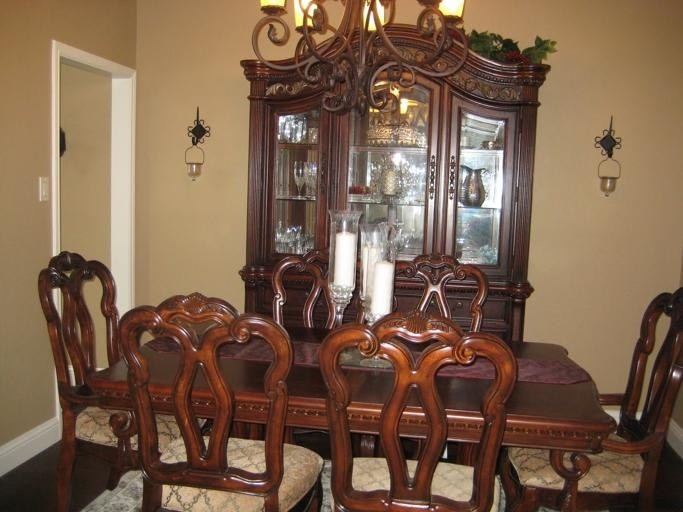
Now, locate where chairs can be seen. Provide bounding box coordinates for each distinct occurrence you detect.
[36,250,209,512]
[316,306,519,512]
[390,253,490,464]
[499,284,682,512]
[244,244,368,444]
[116,289,324,512]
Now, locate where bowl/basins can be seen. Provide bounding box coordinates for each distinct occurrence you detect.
[480,140,500,150]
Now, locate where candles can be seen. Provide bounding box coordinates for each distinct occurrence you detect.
[331,230,356,290]
[361,242,395,323]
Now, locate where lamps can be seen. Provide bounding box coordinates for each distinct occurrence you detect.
[594,112,620,197]
[249,0,469,119]
[179,106,211,183]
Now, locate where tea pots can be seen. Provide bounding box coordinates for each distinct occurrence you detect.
[457,163,495,209]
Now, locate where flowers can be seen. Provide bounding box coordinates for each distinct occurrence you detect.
[451,19,557,65]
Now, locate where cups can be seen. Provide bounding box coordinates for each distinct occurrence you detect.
[275,114,320,144]
[363,213,425,258]
[348,159,425,200]
[459,137,470,149]
[455,236,499,266]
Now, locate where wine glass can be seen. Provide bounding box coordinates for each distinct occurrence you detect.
[272,219,315,255]
[323,207,361,330]
[356,219,404,329]
[291,158,320,198]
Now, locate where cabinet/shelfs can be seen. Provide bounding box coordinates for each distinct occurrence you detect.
[234,20,553,344]
